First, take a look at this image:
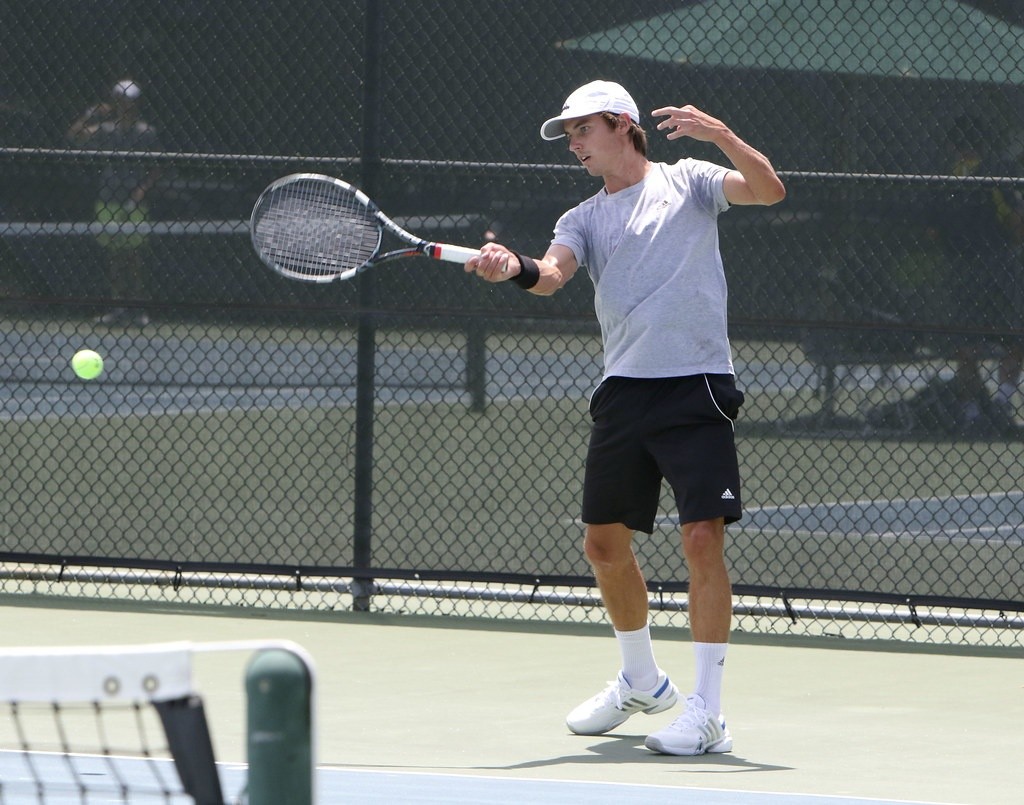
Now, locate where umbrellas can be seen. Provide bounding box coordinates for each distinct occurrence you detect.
[556,0,1024,426]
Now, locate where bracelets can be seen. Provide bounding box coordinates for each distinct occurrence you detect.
[507,250,540,291]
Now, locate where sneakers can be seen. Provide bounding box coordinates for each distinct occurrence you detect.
[645,691,733,757]
[566,669,677,737]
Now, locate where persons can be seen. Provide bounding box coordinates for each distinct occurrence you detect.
[65,79,160,328]
[463,80,785,757]
[885,113,1024,442]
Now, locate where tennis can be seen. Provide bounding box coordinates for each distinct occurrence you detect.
[71,349,105,380]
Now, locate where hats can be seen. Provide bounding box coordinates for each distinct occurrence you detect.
[540,81,640,141]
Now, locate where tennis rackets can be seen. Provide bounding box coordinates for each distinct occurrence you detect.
[249,171,510,283]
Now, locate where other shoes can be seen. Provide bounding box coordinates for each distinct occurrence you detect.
[93,309,121,325]
[135,311,149,327]
